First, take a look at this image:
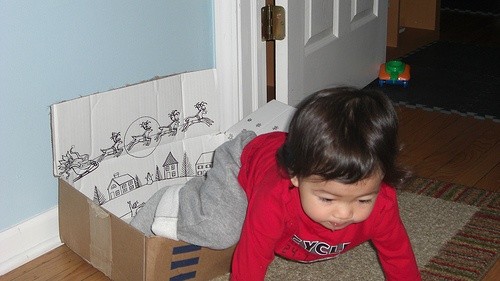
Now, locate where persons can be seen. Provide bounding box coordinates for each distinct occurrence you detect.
[125,85,424,281]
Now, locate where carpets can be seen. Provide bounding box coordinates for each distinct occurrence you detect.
[197,168,500,280]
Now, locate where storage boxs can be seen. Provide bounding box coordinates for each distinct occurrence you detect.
[46,67,301,281]
